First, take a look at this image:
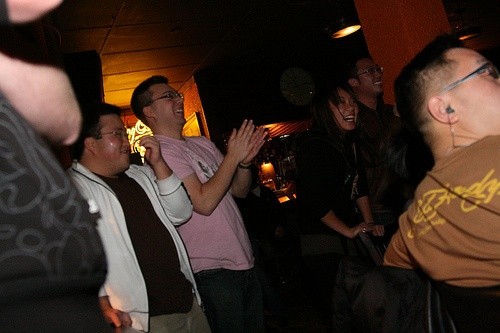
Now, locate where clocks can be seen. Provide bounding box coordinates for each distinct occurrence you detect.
[280,66,316,106]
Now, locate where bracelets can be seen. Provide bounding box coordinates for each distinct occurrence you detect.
[239,162,252,169]
[367,223,375,225]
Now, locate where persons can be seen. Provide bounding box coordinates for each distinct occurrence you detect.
[0,0,431,333]
[379,35,500,287]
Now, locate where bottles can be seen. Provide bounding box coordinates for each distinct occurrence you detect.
[262,173,286,191]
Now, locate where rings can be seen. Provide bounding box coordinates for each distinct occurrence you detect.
[363,228,366,230]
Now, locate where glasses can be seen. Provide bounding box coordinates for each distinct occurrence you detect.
[354,65,385,77]
[437,62,500,96]
[99,129,129,139]
[145,92,184,106]
